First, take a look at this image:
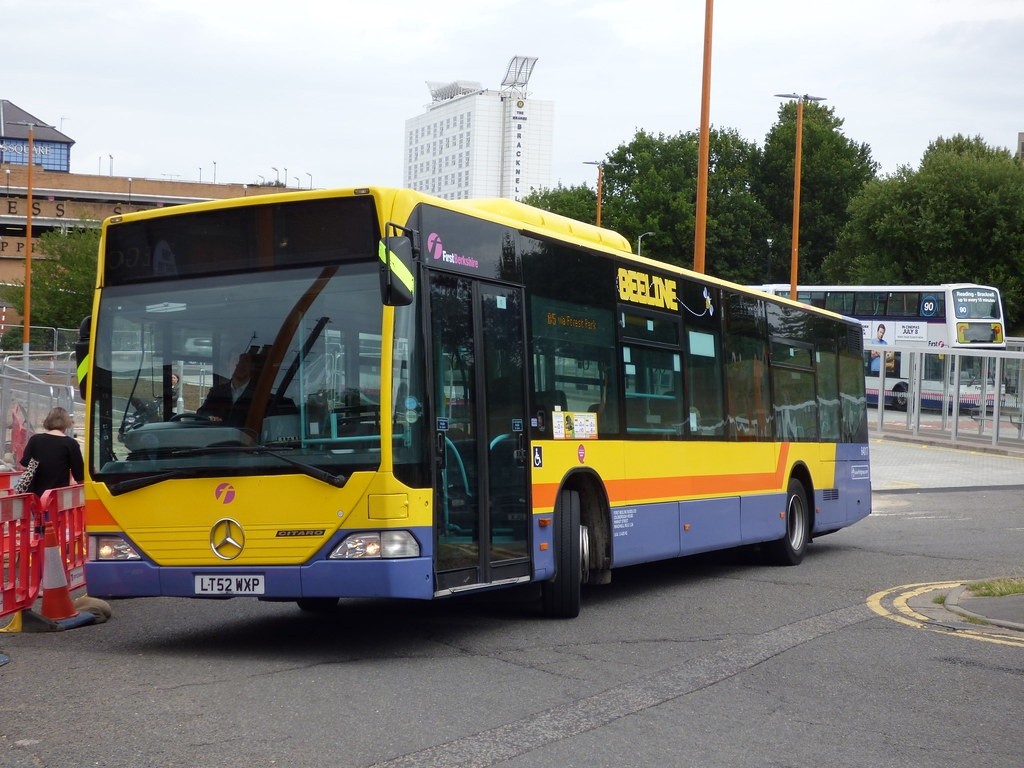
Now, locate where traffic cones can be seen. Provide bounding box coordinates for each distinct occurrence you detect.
[38,519,94,630]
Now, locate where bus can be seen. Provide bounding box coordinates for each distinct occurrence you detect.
[755,282,1007,414]
[302,329,469,413]
[77,191,872,622]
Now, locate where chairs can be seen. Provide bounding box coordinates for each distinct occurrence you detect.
[535,391,567,432]
[588,389,680,429]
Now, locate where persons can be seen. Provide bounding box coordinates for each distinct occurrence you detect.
[19,407,84,598]
[872,324,888,345]
[195,346,280,434]
[172,373,180,407]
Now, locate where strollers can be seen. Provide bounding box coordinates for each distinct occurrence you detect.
[117,395,162,443]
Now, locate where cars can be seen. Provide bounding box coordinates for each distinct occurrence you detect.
[183,337,215,358]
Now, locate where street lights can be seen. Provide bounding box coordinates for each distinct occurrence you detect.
[307,172,314,191]
[284,167,288,186]
[199,167,202,183]
[294,176,301,190]
[272,167,280,185]
[213,161,217,184]
[638,231,656,256]
[128,177,133,209]
[4,120,58,380]
[776,90,828,290]
[98,156,101,174]
[109,154,114,176]
[581,161,620,228]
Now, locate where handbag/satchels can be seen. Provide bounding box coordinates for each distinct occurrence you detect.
[15,456,39,494]
